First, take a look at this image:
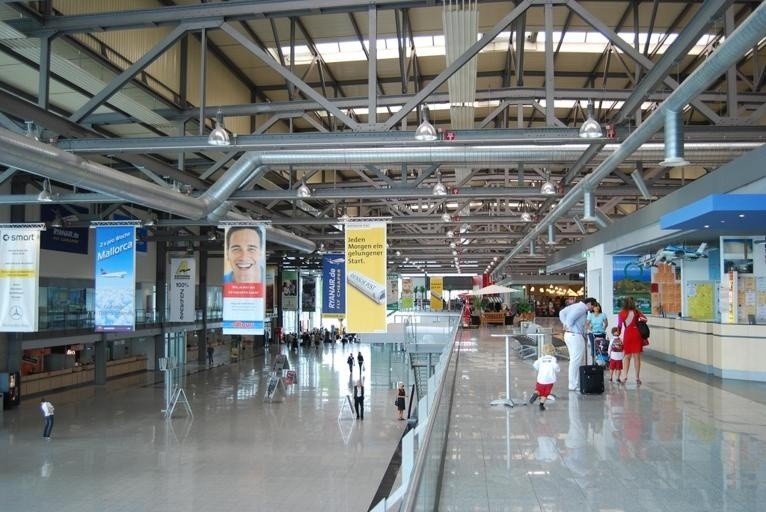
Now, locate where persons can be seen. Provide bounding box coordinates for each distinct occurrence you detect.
[586,303,608,365]
[608,327,624,383]
[353,380,365,421]
[557,297,598,391]
[281,324,356,352]
[39,398,54,440]
[529,344,560,410]
[223,223,264,283]
[205,344,214,366]
[617,298,651,387]
[394,383,408,422]
[357,351,364,373]
[346,353,354,372]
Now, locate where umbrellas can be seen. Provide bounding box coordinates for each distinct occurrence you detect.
[465,284,522,311]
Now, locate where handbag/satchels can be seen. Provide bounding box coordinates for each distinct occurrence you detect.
[637,321,649,339]
[395,401,400,406]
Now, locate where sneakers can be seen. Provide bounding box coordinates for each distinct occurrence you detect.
[540,403,544,410]
[529,393,537,403]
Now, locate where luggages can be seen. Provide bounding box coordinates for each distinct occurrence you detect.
[580,333,604,394]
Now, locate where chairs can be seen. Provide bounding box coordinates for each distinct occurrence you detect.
[511,326,570,361]
